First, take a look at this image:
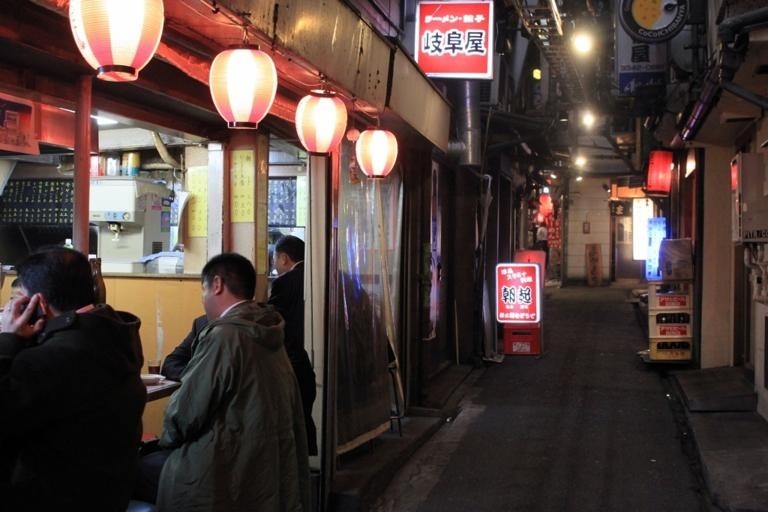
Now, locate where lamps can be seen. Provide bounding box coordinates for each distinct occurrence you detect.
[69,0,164,83]
[356,128,399,180]
[646,150,673,199]
[208,41,277,129]
[295,87,348,157]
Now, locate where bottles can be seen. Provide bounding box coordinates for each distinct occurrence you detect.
[63,238,73,249]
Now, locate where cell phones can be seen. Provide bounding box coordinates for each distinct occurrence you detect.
[19,299,48,326]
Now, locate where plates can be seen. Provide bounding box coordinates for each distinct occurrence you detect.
[141,374,166,384]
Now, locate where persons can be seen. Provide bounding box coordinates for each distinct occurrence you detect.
[536,221,549,269]
[529,223,538,244]
[9,279,26,302]
[160,310,320,458]
[0,248,148,512]
[135,253,314,512]
[271,235,368,347]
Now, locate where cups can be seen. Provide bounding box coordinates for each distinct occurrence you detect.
[147,358,162,375]
